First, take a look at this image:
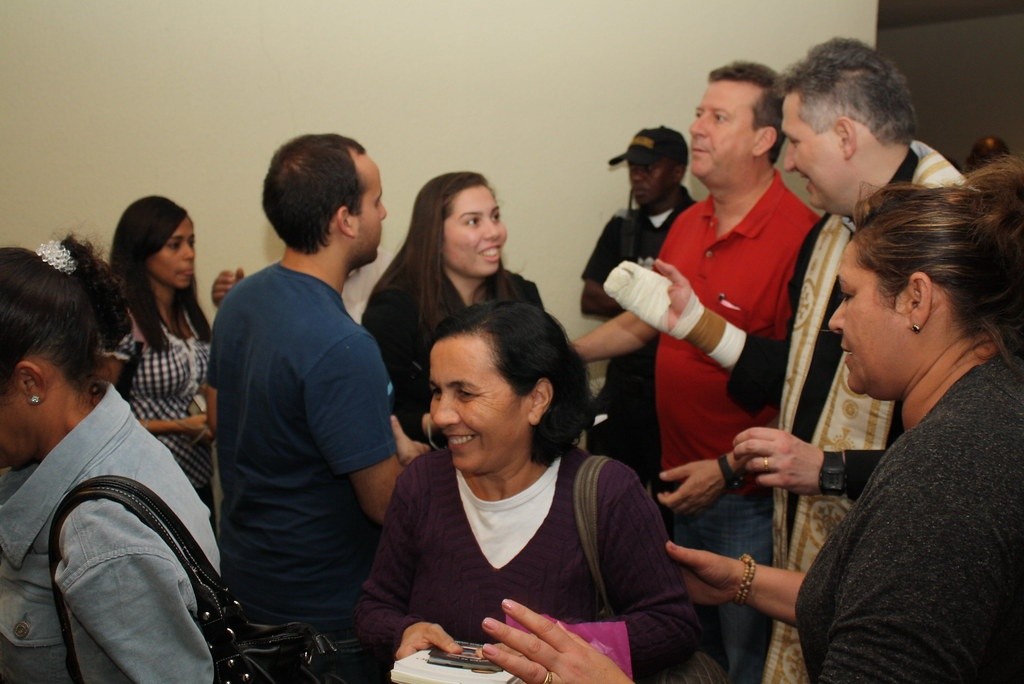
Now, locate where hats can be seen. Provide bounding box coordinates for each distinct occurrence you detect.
[609,126,688,168]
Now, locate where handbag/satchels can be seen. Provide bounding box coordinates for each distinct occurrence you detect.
[573,456,729,684]
[48,476,349,684]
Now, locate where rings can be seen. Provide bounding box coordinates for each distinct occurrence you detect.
[542,671,552,683]
[764,457,769,471]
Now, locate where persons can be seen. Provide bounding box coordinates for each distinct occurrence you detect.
[0,232,221,684]
[667,157,1023,684]
[0,39,1024,684]
[354,300,701,684]
[206,133,407,684]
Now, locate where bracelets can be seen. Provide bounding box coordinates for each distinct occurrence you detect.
[734,553,755,605]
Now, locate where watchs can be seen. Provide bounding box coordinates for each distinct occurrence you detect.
[717,455,748,490]
[818,451,848,497]
